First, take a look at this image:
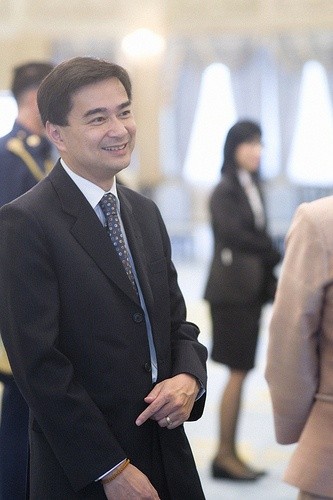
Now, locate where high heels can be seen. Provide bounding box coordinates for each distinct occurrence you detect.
[212,463,266,482]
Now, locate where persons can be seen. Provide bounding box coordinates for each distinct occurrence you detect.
[265,195,333,500]
[0,56,208,500]
[203,121,283,482]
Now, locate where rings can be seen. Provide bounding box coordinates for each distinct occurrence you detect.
[166,416,172,424]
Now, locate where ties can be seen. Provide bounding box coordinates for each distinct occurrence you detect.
[99,192,141,304]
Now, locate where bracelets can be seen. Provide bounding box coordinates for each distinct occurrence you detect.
[100,459,130,483]
